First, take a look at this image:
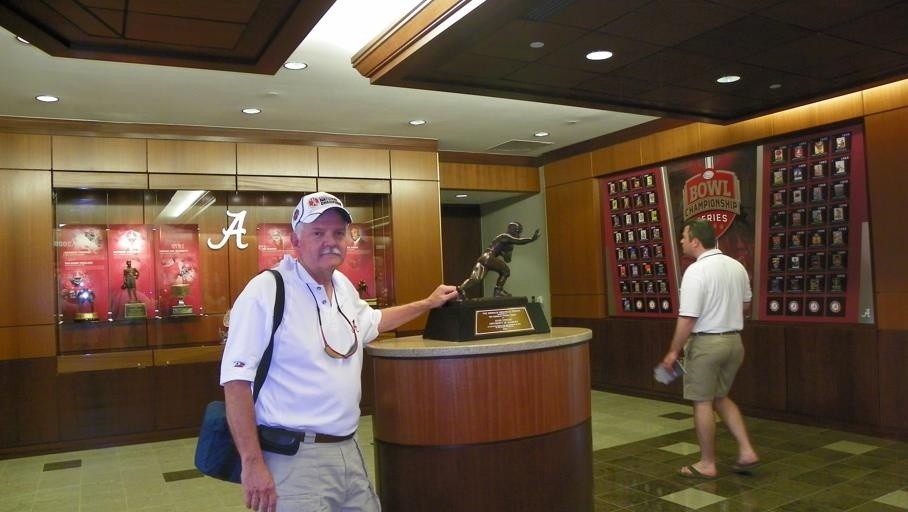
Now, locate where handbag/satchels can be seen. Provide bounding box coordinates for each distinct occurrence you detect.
[193,399,248,485]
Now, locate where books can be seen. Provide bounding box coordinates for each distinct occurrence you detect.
[652,359,688,386]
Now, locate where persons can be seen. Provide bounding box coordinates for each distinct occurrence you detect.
[662,218,760,479]
[123,260,140,303]
[220,191,460,512]
[456,220,542,303]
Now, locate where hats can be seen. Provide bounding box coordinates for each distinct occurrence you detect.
[291,192,353,232]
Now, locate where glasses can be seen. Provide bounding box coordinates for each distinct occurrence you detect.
[325,330,359,359]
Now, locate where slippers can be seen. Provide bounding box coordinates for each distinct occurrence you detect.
[674,461,720,479]
[727,461,763,473]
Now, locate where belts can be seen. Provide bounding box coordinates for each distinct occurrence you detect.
[278,428,362,444]
[689,329,746,337]
